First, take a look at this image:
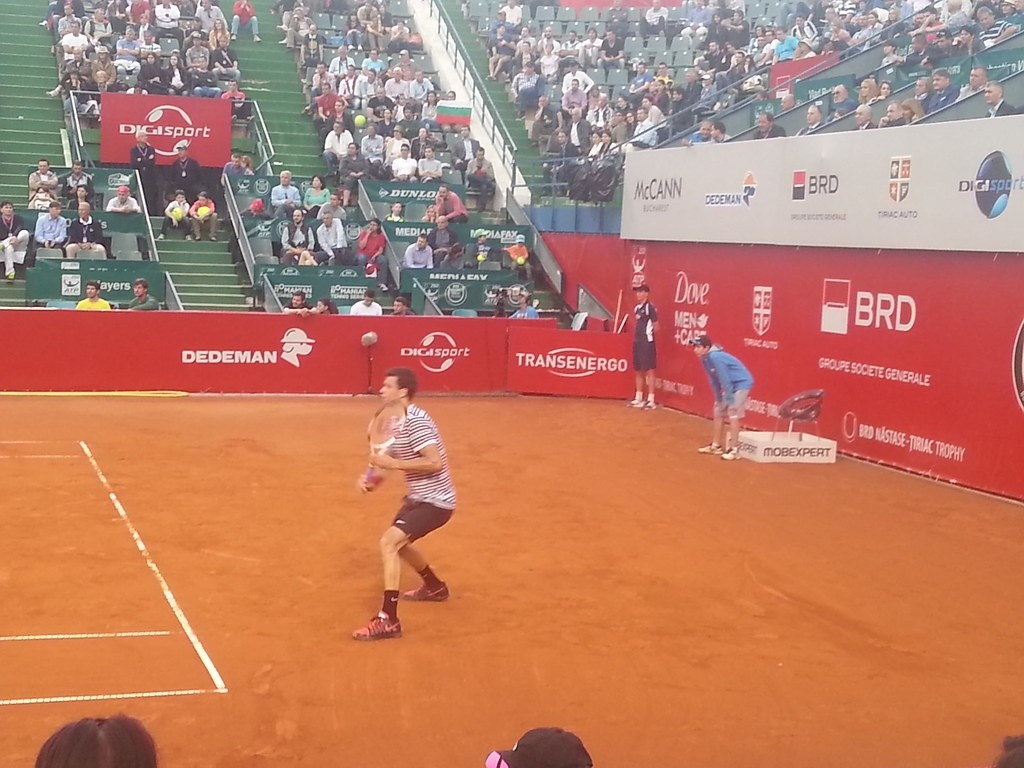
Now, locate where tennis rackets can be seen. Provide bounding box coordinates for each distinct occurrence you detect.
[364,398,409,486]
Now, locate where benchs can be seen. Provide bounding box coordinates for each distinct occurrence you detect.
[150,216,261,312]
[220,0,364,178]
[0,0,70,307]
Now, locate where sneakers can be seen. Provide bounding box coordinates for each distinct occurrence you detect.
[626,400,644,408]
[640,402,656,410]
[353,614,401,641]
[402,581,450,600]
[720,447,743,461]
[697,443,726,453]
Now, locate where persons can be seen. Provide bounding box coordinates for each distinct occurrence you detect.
[36,713,159,768]
[723,0,1024,140]
[238,170,538,317]
[689,335,752,460]
[270,0,496,210]
[488,0,729,201]
[350,367,456,641]
[40,0,259,185]
[1,130,218,309]
[627,284,657,409]
[486,727,593,767]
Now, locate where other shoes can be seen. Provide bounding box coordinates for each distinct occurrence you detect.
[6,271,16,282]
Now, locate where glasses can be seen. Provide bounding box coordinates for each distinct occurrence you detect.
[832,91,840,95]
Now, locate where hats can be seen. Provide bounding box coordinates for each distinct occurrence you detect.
[515,234,525,243]
[568,60,580,67]
[392,126,406,136]
[526,62,536,68]
[633,284,650,293]
[473,229,489,238]
[495,727,592,768]
[117,185,130,194]
[175,144,186,151]
[400,50,409,55]
[689,334,712,348]
[798,38,814,50]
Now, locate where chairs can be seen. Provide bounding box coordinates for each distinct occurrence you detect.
[54,0,237,116]
[771,388,824,441]
[300,0,497,184]
[242,186,545,316]
[471,0,1023,141]
[37,186,162,311]
